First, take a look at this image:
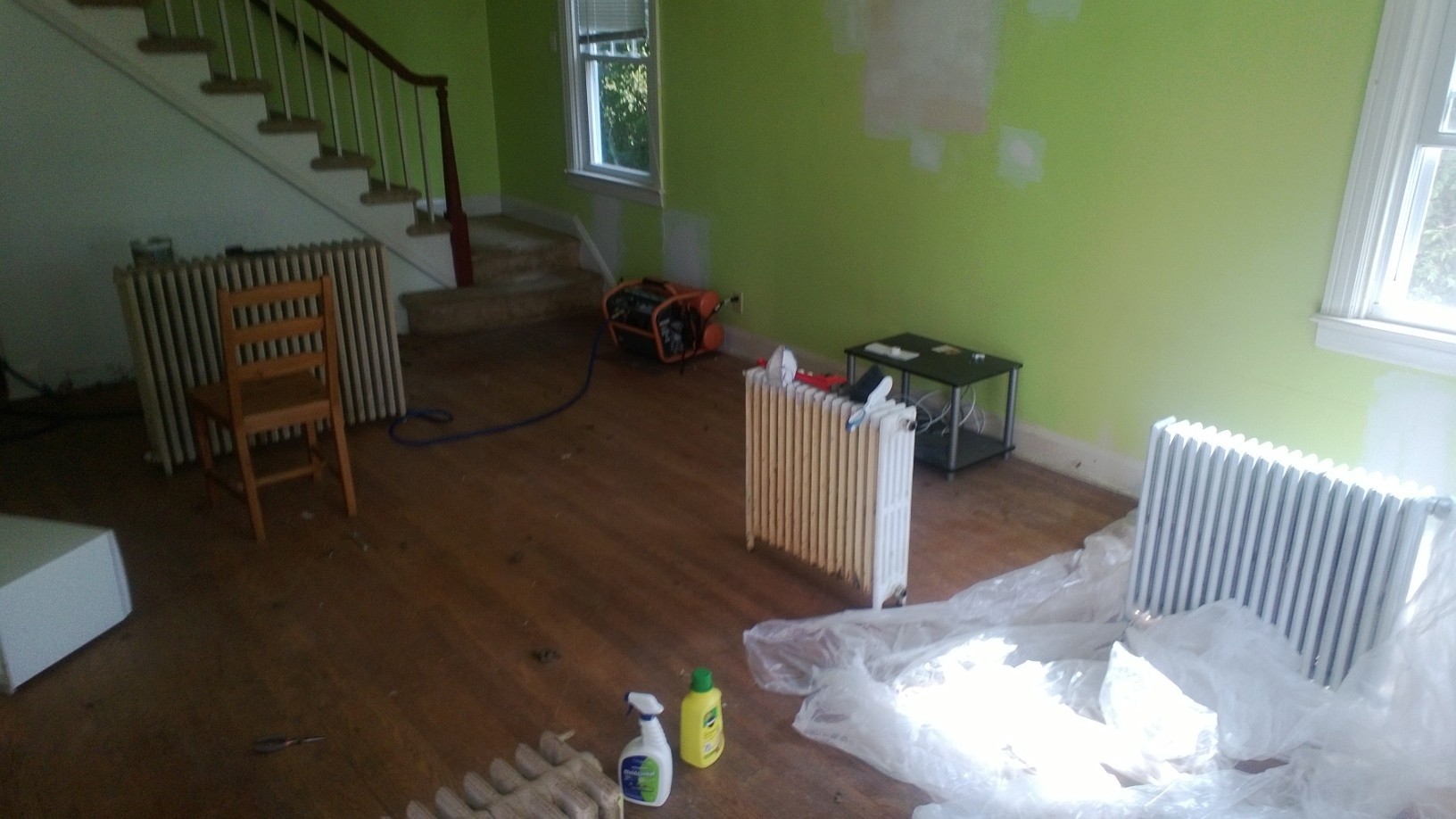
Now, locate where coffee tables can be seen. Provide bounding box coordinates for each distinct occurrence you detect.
[845,331,1025,482]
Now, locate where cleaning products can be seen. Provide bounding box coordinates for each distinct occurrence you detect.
[680,668,725,768]
[616,691,674,807]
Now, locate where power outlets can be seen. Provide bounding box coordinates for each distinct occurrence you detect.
[728,287,747,314]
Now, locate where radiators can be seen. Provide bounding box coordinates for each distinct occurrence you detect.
[733,358,922,618]
[1122,414,1449,714]
[109,234,413,481]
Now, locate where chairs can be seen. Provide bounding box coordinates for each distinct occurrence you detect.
[182,272,360,542]
